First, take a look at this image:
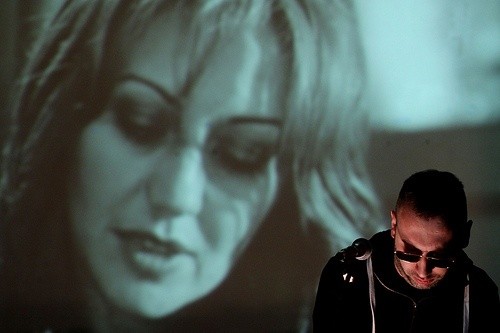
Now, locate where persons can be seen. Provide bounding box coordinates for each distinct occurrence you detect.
[1,0,390,333]
[309,169,500,333]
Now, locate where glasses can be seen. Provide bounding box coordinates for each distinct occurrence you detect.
[394,225,456,269]
